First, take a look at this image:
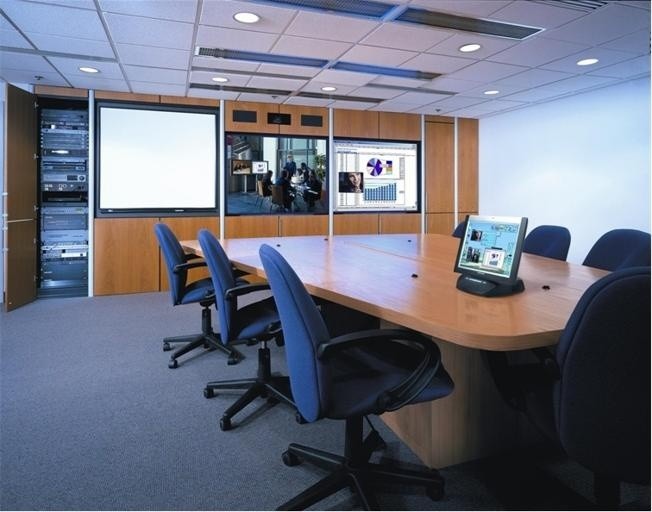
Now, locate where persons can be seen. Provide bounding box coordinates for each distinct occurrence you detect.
[344,172,363,192]
[472,231,482,240]
[262,156,321,212]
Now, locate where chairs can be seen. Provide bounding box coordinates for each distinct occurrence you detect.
[518,267,650,511]
[153,222,257,368]
[198,227,310,432]
[582,228,651,273]
[524,225,572,260]
[255,179,322,215]
[453,222,466,238]
[257,245,454,511]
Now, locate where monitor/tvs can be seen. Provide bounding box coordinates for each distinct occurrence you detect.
[224,130,329,217]
[453,215,528,297]
[95,101,220,218]
[332,136,422,214]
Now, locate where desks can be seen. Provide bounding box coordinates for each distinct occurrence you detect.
[174,233,616,469]
